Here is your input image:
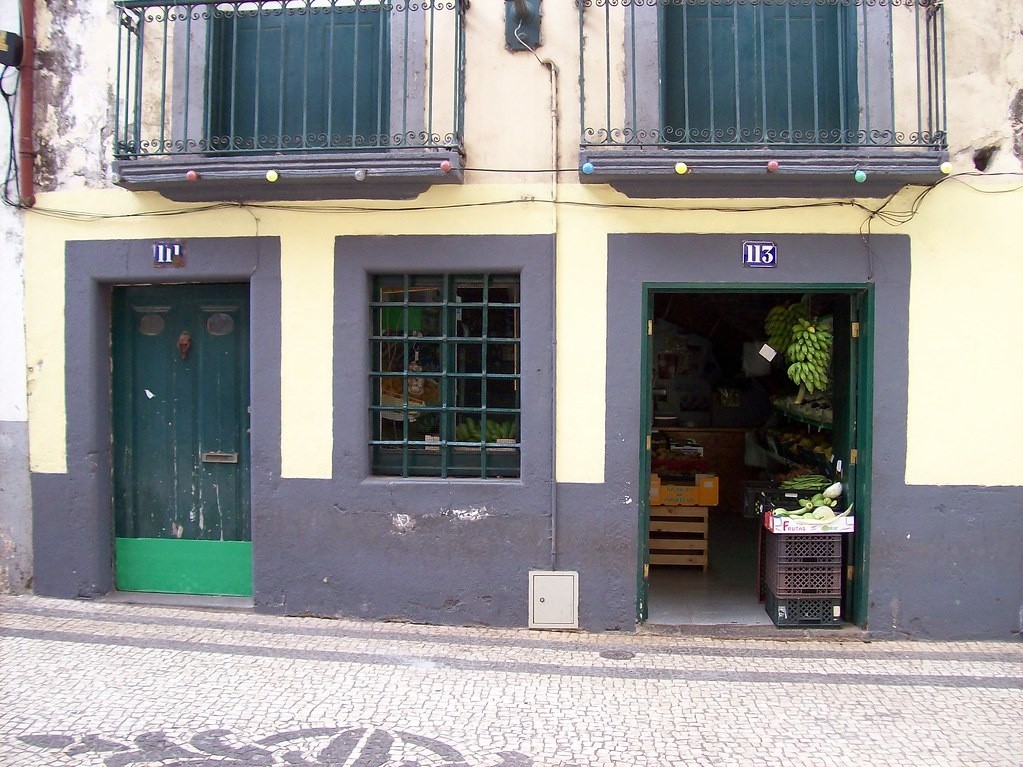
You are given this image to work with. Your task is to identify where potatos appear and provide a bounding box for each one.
[651,447,704,461]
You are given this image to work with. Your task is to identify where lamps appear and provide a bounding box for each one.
[177,330,193,361]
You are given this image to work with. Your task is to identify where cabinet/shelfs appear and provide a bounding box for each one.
[761,412,834,476]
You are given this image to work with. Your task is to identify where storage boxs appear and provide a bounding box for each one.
[651,438,709,480]
[765,432,833,474]
[762,512,855,630]
[736,481,843,519]
[650,472,719,507]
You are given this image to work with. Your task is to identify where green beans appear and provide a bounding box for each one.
[778,476,829,491]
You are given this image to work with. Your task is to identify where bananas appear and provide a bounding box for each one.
[765,304,833,394]
[453,416,517,440]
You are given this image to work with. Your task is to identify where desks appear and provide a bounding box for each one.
[653,426,753,499]
